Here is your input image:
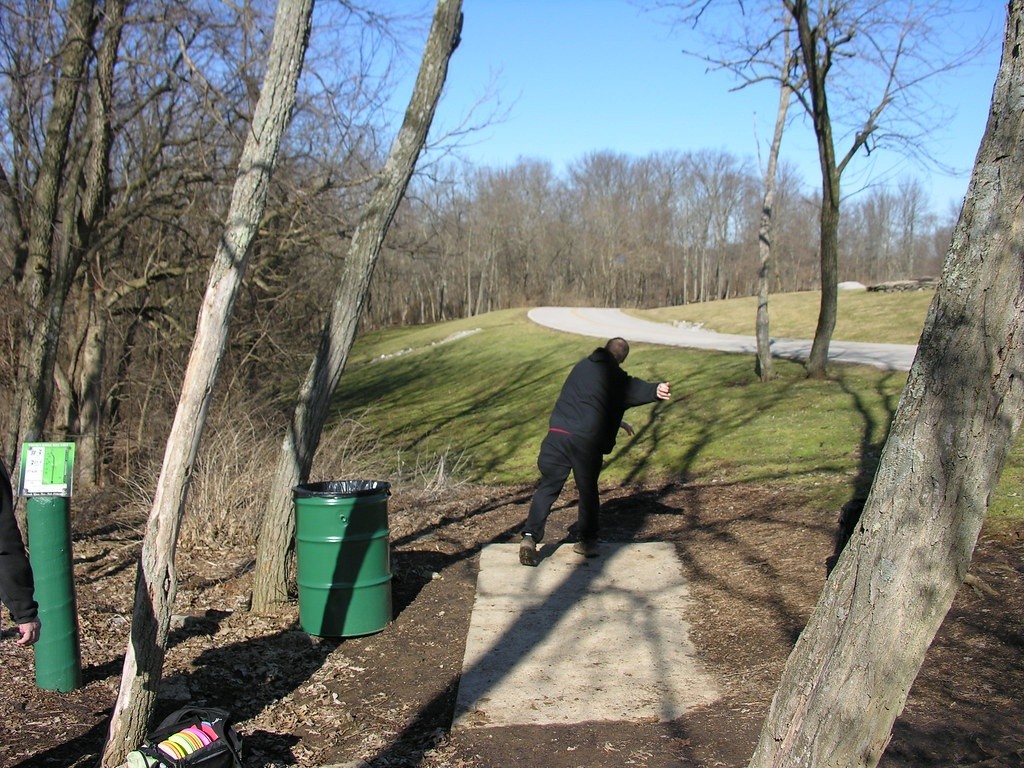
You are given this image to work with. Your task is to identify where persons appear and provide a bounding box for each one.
[0,459,42,646]
[520,338,671,567]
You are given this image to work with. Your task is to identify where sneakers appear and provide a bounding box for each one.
[519,536,538,567]
[572,542,601,559]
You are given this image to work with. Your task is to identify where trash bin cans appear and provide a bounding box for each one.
[293,480,394,636]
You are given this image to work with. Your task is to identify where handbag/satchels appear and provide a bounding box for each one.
[126,705,243,768]
